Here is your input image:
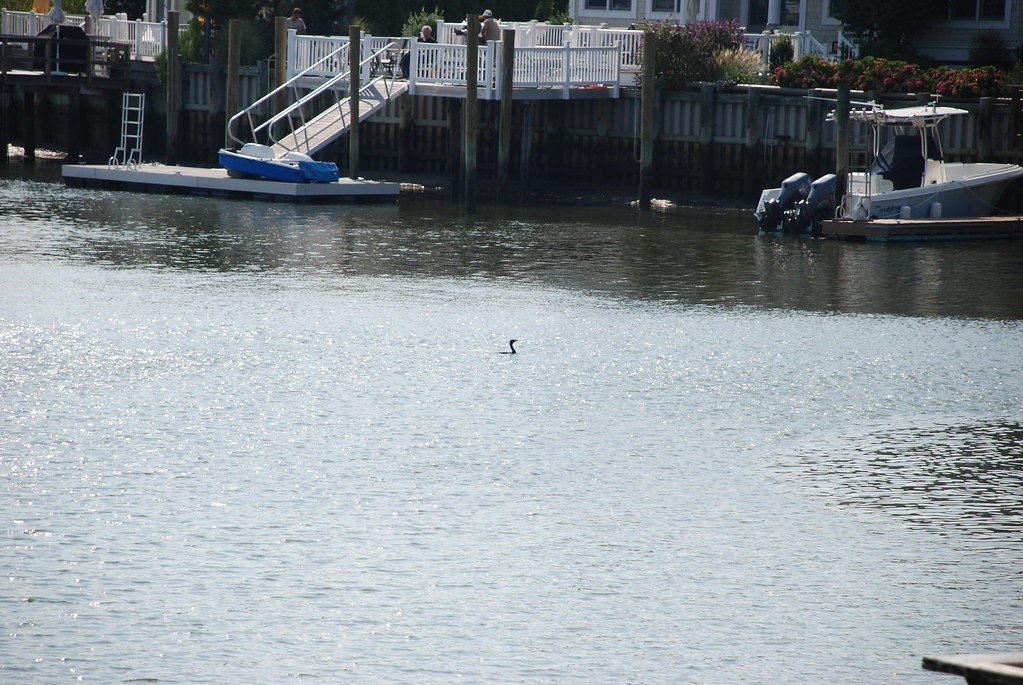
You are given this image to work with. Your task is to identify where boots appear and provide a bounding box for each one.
[453,28,462,35]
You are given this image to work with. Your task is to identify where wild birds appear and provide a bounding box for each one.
[498,339,519,354]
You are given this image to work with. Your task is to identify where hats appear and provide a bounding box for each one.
[482,9,491,16]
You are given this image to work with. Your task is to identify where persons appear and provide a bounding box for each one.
[286,7,306,34]
[398,24,440,81]
[481,9,501,41]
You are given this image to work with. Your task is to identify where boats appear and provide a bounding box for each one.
[755,105,1022,218]
[217,142,340,183]
